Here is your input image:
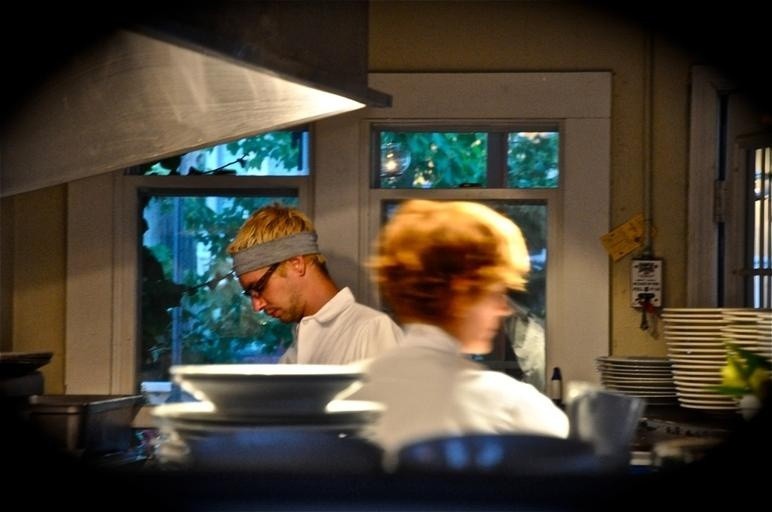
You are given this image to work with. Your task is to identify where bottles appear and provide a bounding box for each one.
[551,367,561,404]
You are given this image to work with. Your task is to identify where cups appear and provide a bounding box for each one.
[564,379,648,465]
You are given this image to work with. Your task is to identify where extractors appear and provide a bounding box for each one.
[0,1,392,201]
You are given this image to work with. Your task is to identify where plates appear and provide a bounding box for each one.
[150,363,386,473]
[595,307,772,411]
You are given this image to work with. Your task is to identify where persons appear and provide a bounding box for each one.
[336,198,571,472]
[226,202,404,365]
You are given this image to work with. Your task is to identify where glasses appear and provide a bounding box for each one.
[242,263,280,297]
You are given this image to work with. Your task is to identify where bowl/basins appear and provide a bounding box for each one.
[398,434,595,512]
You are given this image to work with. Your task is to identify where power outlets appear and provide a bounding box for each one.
[631,283,664,309]
